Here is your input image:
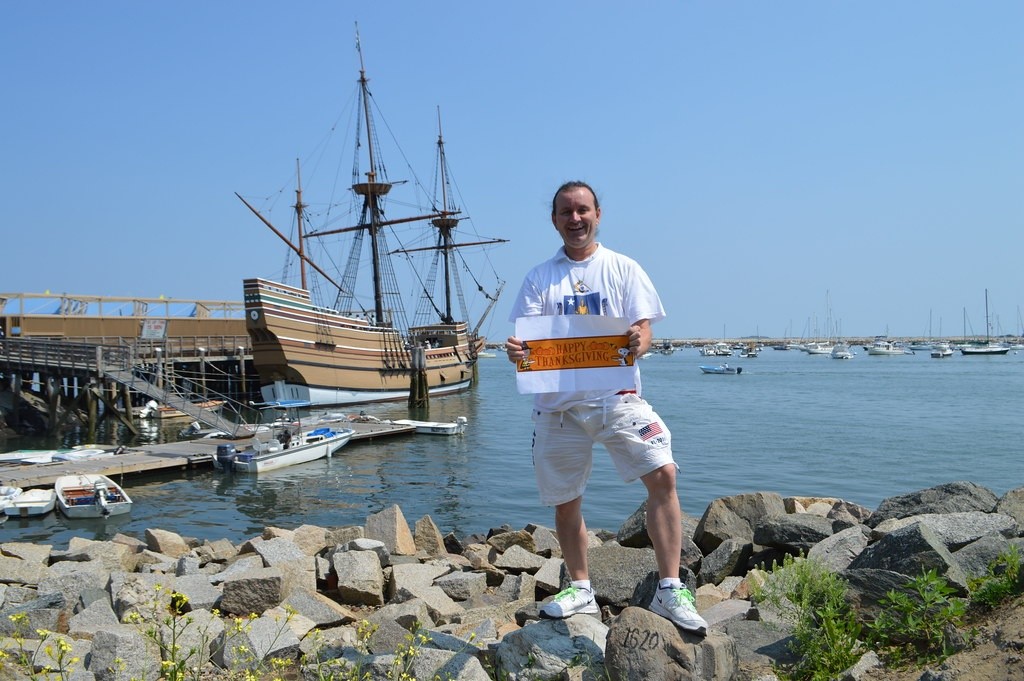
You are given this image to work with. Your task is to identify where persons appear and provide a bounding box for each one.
[507,180,708,635]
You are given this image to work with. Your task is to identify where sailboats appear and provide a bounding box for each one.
[771,289,854,359]
[863,289,1024,357]
[234,20,509,409]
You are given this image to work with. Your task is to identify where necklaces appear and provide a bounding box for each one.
[564,255,594,293]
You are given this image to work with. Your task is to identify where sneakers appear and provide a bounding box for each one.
[648,582,707,637]
[538,587,598,619]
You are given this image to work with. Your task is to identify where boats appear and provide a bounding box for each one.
[210,400,356,473]
[699,342,764,358]
[0,486,23,513]
[699,366,742,375]
[4,488,56,515]
[55,474,133,518]
[270,413,469,435]
[0,449,114,463]
